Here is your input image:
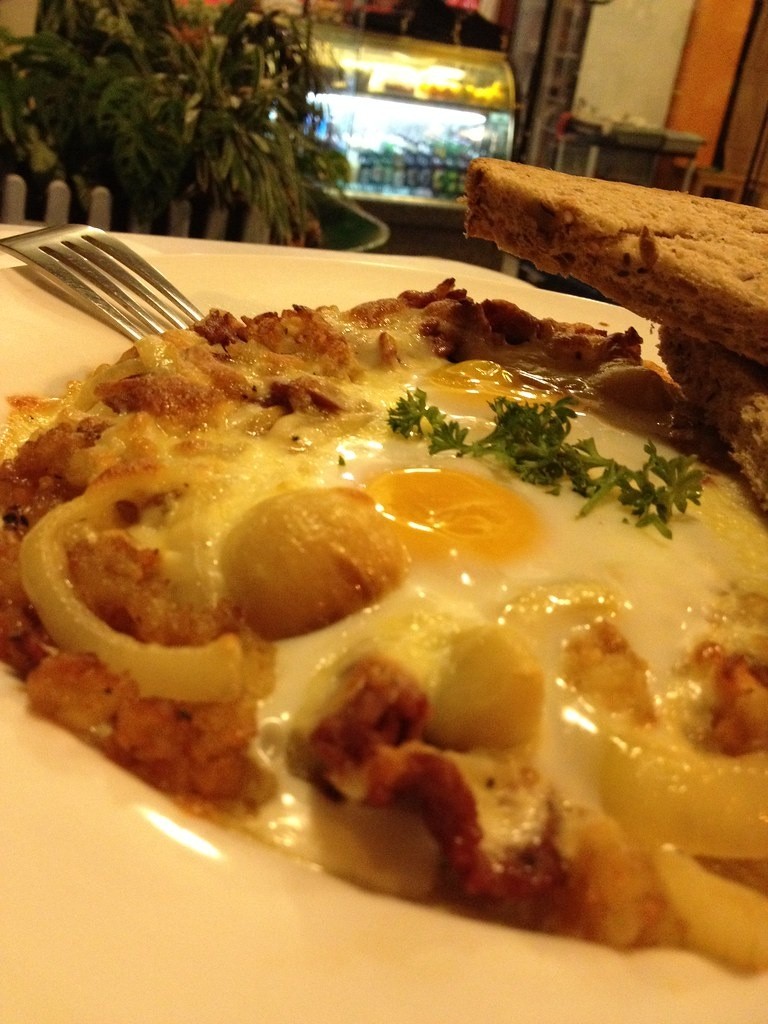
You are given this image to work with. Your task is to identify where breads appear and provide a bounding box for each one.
[464,154,768,517]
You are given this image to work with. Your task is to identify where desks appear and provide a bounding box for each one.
[556,117,707,193]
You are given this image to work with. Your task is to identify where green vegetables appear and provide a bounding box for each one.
[380,385,711,541]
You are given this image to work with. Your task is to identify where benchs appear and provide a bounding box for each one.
[669,161,747,203]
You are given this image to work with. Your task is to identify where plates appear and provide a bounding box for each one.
[0,237,767,1024]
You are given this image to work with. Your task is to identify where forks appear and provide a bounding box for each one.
[0,221,206,342]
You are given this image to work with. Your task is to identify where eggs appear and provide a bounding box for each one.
[361,460,567,587]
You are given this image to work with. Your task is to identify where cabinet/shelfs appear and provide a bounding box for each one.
[245,11,517,270]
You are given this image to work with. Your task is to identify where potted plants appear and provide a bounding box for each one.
[0,0,394,256]
